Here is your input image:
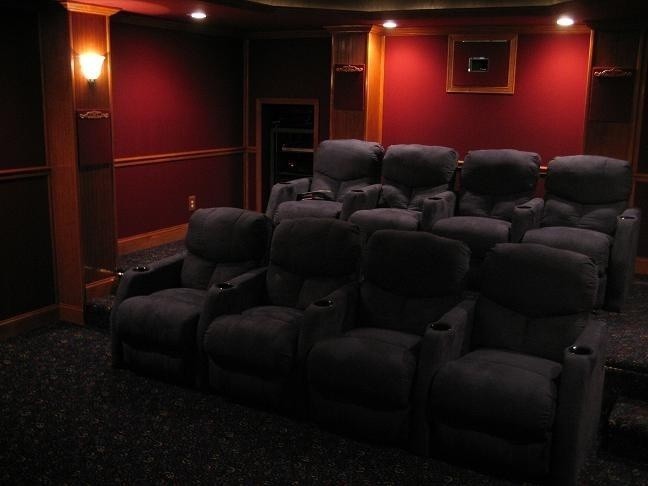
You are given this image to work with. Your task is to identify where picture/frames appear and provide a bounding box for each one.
[446,31,518,94]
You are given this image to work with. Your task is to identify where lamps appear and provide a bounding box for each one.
[77,52,105,88]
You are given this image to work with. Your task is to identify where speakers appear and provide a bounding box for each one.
[333,64,365,113]
[588,66,633,122]
